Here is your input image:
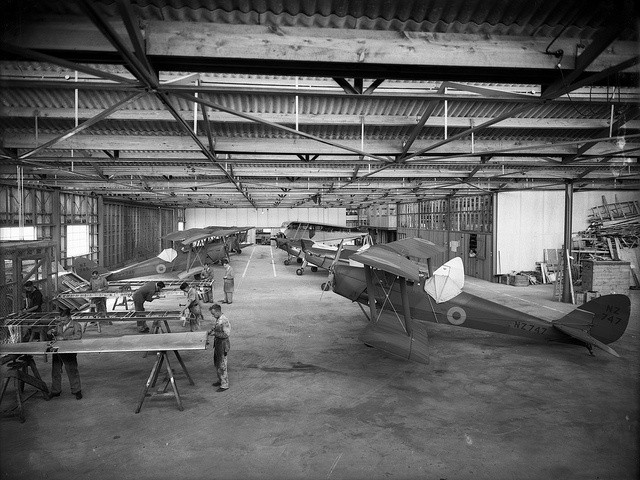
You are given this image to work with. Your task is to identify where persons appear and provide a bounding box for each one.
[48,307,83,400]
[86,271,110,324]
[206,304,231,392]
[222,259,234,305]
[200,263,214,304]
[22,280,44,341]
[178,283,203,333]
[132,281,166,333]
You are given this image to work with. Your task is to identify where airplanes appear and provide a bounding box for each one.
[271,221,374,275]
[72,228,228,281]
[160,226,253,255]
[328,237,631,365]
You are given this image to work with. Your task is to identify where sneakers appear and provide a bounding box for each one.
[217,387,229,392]
[48,392,61,399]
[213,382,221,386]
[76,390,82,400]
